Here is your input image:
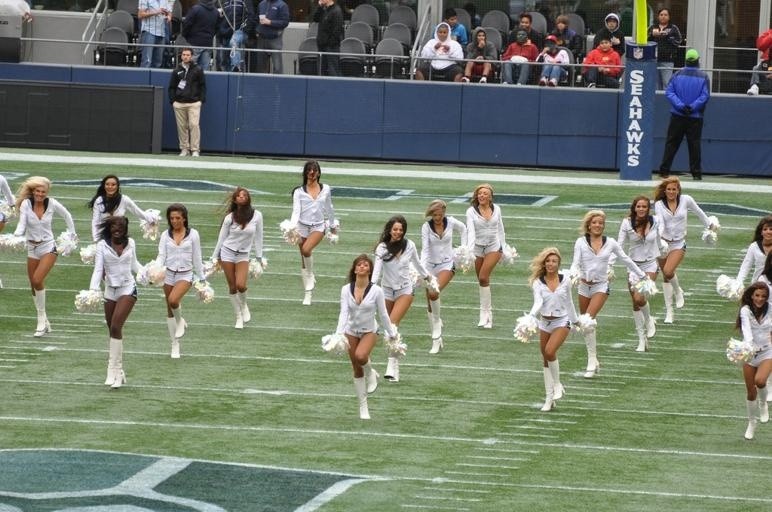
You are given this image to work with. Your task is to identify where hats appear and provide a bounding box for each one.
[684,48,700,62]
[543,35,559,45]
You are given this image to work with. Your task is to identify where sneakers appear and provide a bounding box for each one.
[746,83,759,95]
[459,76,560,87]
[177,150,201,157]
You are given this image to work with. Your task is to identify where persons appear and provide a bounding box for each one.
[79,176,161,280]
[321,184,520,420]
[512,175,721,412]
[280,161,340,305]
[415,0,683,91]
[168,47,207,157]
[311,0,343,77]
[203,187,266,330]
[747,29,772,95]
[149,205,215,359]
[75,214,149,388]
[660,49,710,180]
[0,174,17,288]
[135,0,290,73]
[715,216,772,440]
[0,176,78,337]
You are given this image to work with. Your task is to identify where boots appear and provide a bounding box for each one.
[638,300,656,338]
[667,274,684,309]
[428,297,443,340]
[477,285,493,329]
[546,359,565,401]
[662,282,673,324]
[540,366,556,412]
[353,375,371,420]
[582,324,600,379]
[361,357,380,395]
[757,386,769,423]
[31,254,317,388]
[383,354,399,382]
[744,398,757,440]
[427,310,444,354]
[632,308,649,352]
